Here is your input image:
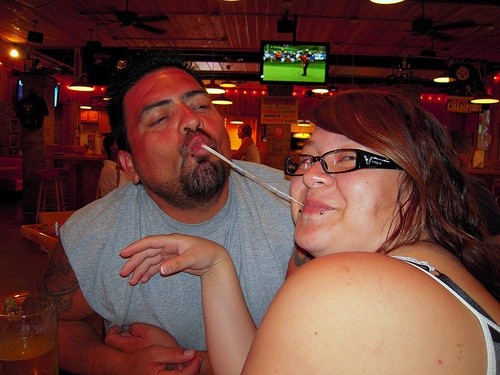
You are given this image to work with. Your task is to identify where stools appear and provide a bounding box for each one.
[33,168,65,225]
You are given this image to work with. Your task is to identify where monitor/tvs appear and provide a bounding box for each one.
[259,40,329,85]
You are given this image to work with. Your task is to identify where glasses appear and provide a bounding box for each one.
[283,148,411,176]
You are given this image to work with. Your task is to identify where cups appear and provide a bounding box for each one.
[0,294,59,375]
[50,217,65,238]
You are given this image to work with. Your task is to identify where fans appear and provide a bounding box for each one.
[401,0,478,43]
[89,0,171,35]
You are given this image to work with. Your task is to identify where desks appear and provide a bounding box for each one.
[41,151,105,210]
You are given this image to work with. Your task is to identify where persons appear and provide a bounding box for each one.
[263,47,326,64]
[114,83,500,375]
[94,133,136,204]
[298,51,310,76]
[228,123,260,164]
[14,45,330,374]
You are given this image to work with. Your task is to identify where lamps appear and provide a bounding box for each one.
[205,71,236,105]
[66,47,95,91]
[433,60,456,84]
[470,63,500,104]
